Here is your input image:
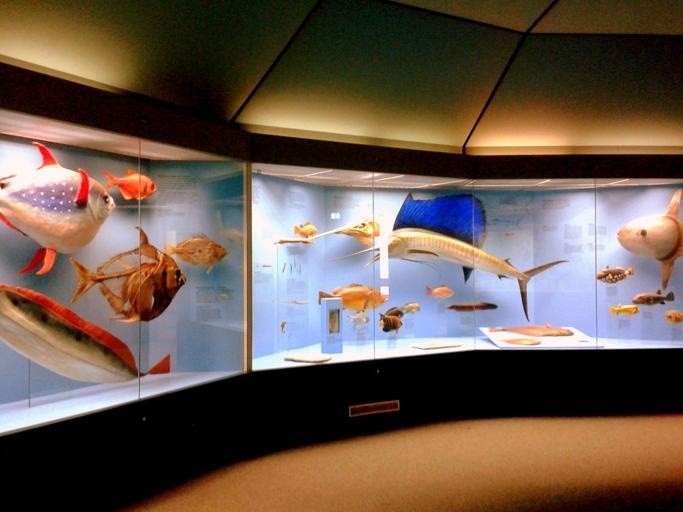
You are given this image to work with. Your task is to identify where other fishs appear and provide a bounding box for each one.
[1,141,683,382]
